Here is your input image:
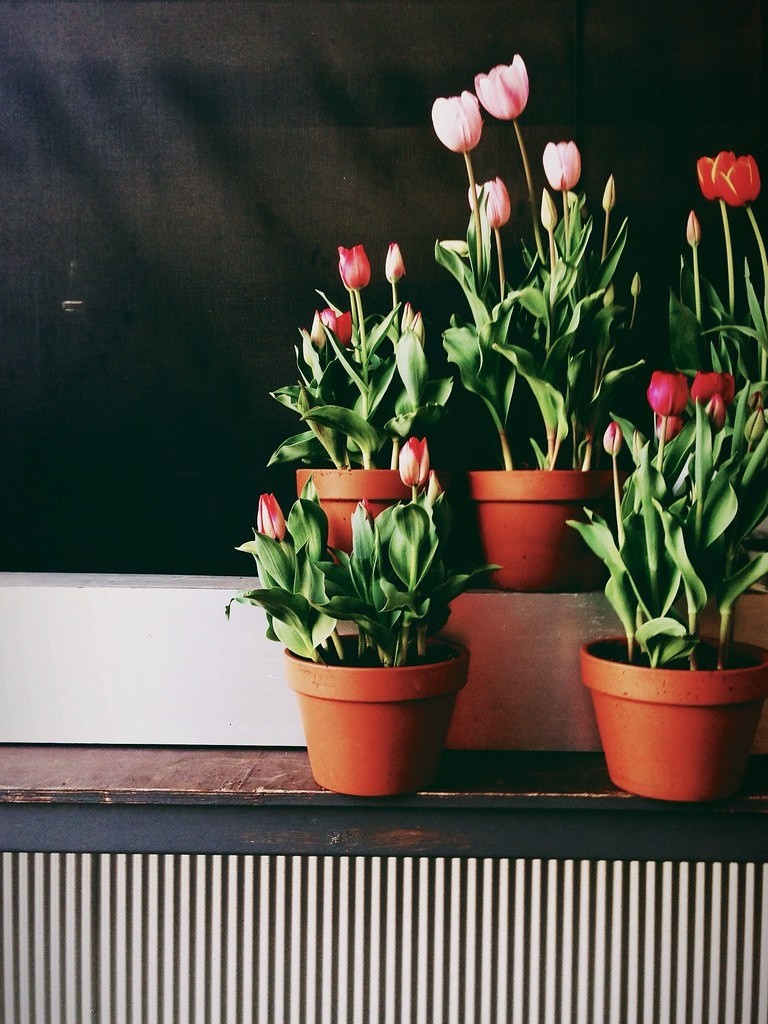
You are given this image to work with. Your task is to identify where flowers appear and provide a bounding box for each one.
[659,152,767,381]
[562,370,768,670]
[225,436,501,667]
[430,55,643,472]
[266,242,452,469]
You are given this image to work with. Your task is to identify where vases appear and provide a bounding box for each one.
[468,471,628,592]
[578,633,767,801]
[282,632,470,796]
[295,469,427,565]
